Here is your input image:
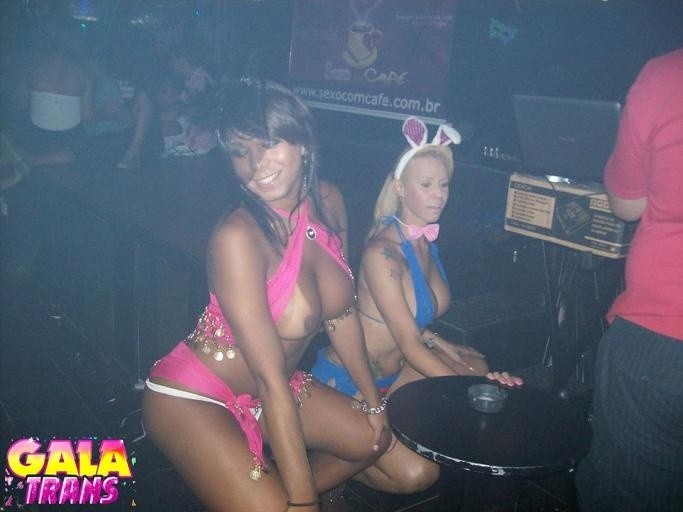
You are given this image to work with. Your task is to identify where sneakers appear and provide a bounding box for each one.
[113,147,144,171]
[166,141,210,157]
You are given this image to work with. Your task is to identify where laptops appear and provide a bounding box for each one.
[510,91,623,188]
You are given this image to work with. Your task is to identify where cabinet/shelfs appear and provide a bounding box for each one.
[501,226,630,369]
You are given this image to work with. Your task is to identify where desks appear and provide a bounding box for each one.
[385,375,593,512]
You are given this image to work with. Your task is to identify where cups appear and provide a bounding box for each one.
[349,21,383,59]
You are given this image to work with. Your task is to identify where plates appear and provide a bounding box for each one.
[341,49,379,68]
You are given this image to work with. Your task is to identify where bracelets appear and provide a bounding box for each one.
[427,330,440,347]
[285,499,322,508]
[357,393,390,420]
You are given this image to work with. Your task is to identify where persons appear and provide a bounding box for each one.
[309,108,523,497]
[139,74,400,512]
[0,0,222,176]
[572,46,683,512]
